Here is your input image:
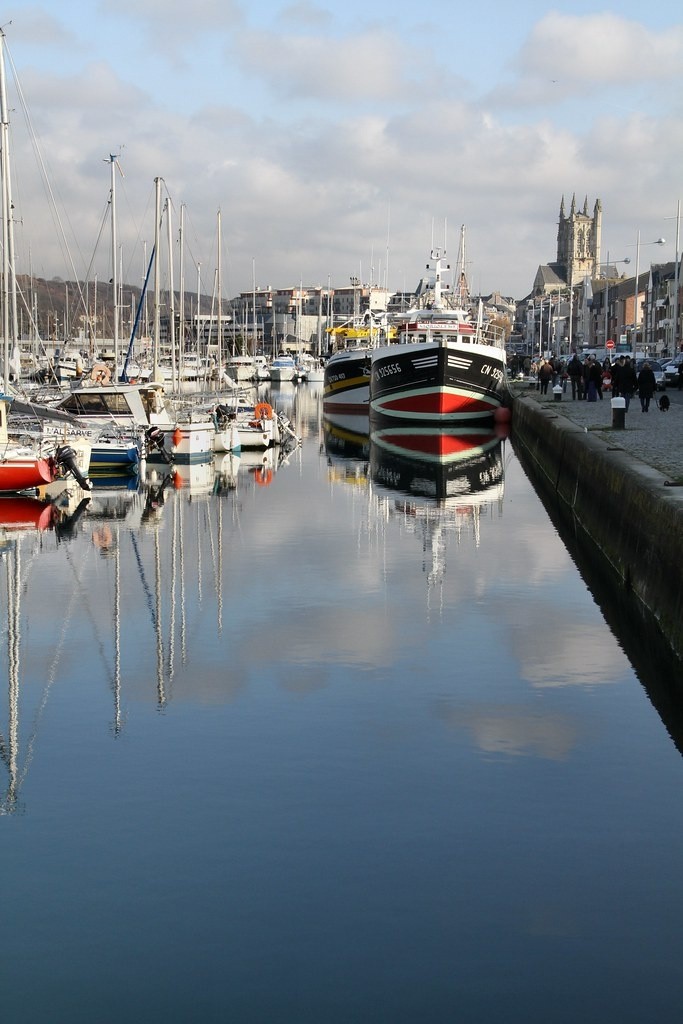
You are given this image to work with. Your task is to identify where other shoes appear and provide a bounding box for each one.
[645,409,648,412]
[642,406,646,412]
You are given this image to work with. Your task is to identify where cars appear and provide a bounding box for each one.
[634,359,666,392]
[558,355,574,365]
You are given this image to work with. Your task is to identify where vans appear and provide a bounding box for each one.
[598,353,649,367]
[657,352,683,386]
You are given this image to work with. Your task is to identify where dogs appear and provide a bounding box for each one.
[659,395,670,412]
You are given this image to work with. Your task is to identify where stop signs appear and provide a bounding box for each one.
[606,340,614,349]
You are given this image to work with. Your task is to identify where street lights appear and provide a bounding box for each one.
[578,267,606,348]
[526,279,582,356]
[595,251,632,349]
[626,230,666,358]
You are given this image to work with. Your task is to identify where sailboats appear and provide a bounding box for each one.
[1,448,284,816]
[0,21,319,515]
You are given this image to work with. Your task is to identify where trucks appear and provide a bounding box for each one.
[579,349,616,365]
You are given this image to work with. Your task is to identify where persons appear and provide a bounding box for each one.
[206,403,229,434]
[506,352,569,395]
[567,353,657,413]
[427,300,432,310]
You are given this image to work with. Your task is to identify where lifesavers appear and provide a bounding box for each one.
[173,429,182,447]
[255,469,273,485]
[90,364,111,385]
[255,403,273,419]
[174,470,182,489]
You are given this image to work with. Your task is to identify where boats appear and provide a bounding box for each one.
[369,416,507,622]
[321,192,396,407]
[368,217,509,424]
[320,404,389,585]
